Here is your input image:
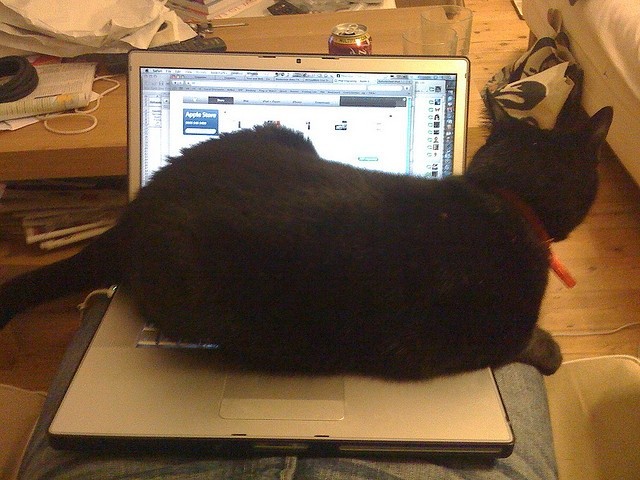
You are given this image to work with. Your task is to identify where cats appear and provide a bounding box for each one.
[2,87,613,383]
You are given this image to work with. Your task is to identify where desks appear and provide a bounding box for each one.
[0,5,450,180]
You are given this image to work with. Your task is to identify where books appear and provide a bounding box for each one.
[165,0,260,23]
[0,61,96,123]
[2,178,128,251]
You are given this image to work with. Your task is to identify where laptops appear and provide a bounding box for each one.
[42,49,530,466]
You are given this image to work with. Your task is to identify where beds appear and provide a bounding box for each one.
[522,0,640,188]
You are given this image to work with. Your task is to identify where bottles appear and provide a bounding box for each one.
[327,21,372,55]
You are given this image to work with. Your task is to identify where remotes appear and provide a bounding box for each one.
[103,36,228,71]
